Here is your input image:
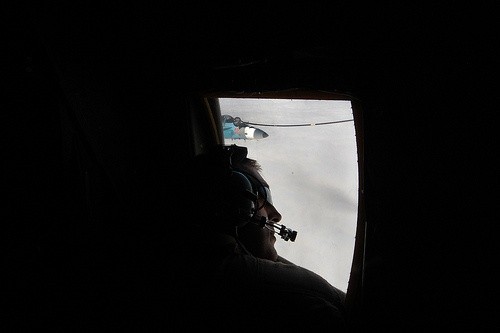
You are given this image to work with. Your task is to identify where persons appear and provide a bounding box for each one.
[167,149,346,333]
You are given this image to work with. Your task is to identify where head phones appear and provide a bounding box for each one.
[214,144,255,223]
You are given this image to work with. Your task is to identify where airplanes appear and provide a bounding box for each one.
[222,115,269,142]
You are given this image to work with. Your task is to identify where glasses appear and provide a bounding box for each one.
[255,186,274,207]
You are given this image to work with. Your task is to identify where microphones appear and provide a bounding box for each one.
[252,214,297,243]
